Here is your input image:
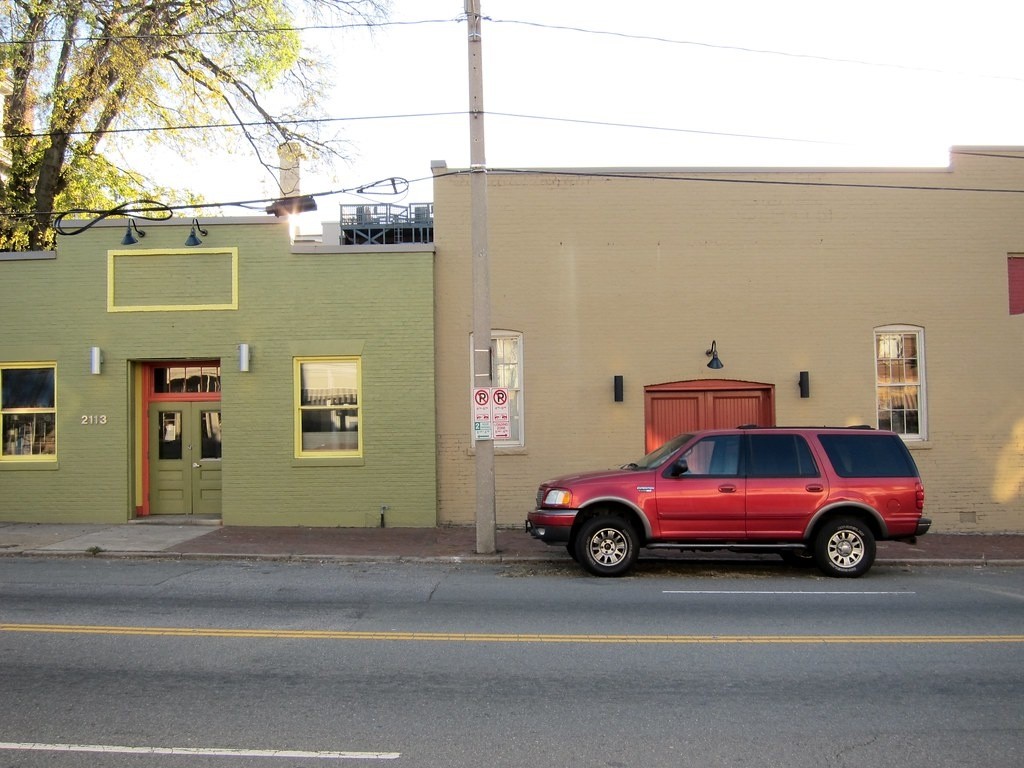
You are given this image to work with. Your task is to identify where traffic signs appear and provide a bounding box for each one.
[473,386,493,440]
[490,387,511,439]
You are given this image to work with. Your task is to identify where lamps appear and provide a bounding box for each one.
[239,343,251,371]
[121,218,145,246]
[614,376,624,402]
[799,371,809,398]
[706,340,724,369]
[185,218,208,246]
[91,346,103,374]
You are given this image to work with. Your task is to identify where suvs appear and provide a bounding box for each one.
[524,424,932,578]
